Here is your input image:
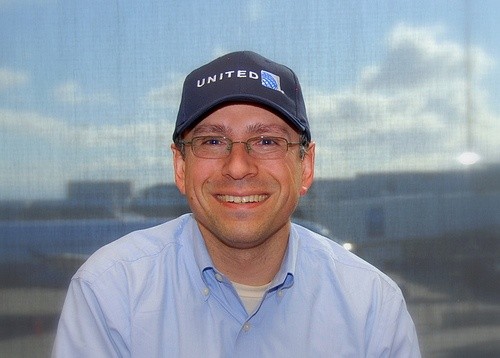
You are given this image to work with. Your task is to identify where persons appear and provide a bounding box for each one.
[52,49,422,358]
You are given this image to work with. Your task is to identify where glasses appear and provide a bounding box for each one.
[175,135,307,159]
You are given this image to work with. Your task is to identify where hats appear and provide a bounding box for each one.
[171,50,314,144]
